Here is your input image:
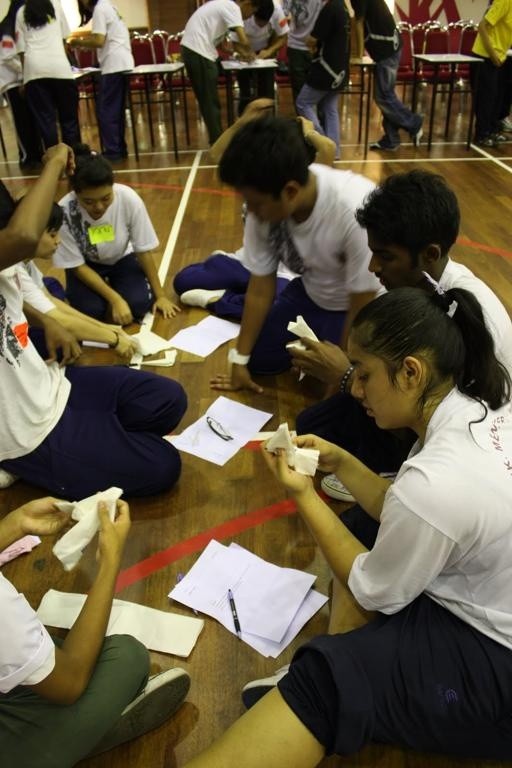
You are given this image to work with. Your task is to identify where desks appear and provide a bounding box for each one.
[126,63,192,161]
[74,67,104,159]
[220,58,279,128]
[412,53,486,152]
[347,57,378,160]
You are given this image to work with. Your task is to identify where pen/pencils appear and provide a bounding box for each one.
[228,589,242,640]
[134,318,146,325]
[113,363,138,367]
[177,573,199,615]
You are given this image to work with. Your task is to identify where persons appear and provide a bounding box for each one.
[0,0,512,172]
[167,97,511,504]
[2,146,189,500]
[0,472,191,766]
[178,287,511,766]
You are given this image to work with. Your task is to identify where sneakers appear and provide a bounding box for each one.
[475,135,499,147]
[86,666,192,758]
[180,289,226,309]
[369,140,401,152]
[491,131,511,144]
[240,662,296,708]
[103,147,129,162]
[320,465,405,505]
[411,116,424,147]
[498,116,512,128]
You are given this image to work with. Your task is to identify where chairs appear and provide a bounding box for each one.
[215,41,291,87]
[364,19,479,112]
[64,30,192,126]
